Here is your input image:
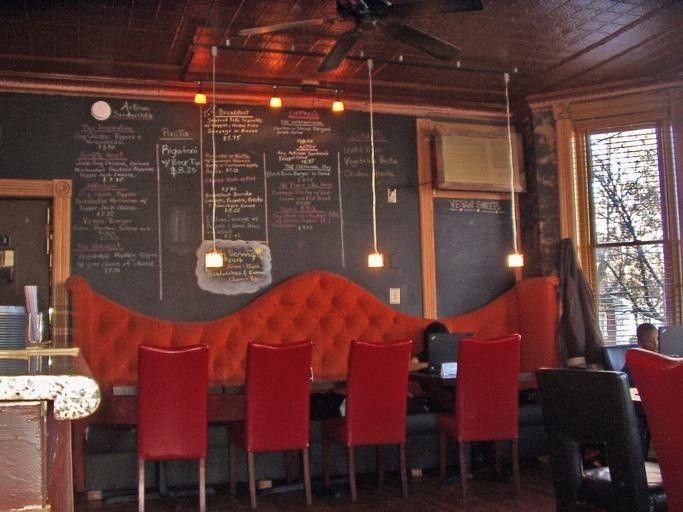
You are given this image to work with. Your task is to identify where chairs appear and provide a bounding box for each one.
[439,336,521,504]
[136,341,211,512]
[225,338,313,512]
[536,343,682,511]
[322,337,417,502]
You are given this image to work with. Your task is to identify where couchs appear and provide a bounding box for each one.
[66,276,562,495]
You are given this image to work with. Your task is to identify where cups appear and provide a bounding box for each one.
[24,313,43,345]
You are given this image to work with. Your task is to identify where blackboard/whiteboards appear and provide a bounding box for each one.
[0,79,523,320]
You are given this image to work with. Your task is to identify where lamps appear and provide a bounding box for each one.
[201,46,227,272]
[501,71,525,270]
[364,55,389,270]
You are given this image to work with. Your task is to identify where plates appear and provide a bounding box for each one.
[0,304,26,377]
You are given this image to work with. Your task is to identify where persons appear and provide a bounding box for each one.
[409,322,511,479]
[620,323,660,463]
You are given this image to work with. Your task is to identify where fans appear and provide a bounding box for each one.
[240,1,486,75]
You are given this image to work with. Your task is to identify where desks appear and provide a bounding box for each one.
[71,370,532,512]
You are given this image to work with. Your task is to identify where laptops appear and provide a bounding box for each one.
[422,332,474,373]
[658,325,683,357]
